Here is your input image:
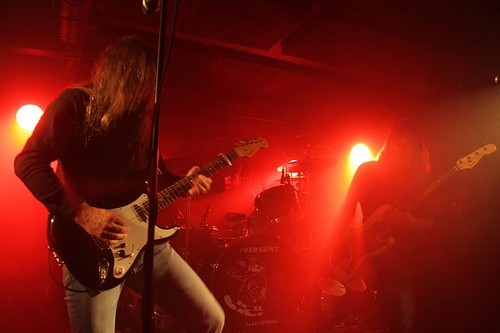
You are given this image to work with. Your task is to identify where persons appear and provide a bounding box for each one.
[325,121,439,330]
[13,33,226,333]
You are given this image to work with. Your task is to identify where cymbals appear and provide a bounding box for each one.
[155,156,237,199]
[275,156,339,173]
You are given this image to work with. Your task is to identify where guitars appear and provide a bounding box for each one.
[46,135,267,291]
[327,144,497,282]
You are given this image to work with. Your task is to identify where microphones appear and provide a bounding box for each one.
[280,167,285,184]
[202,205,211,225]
[142,0,161,14]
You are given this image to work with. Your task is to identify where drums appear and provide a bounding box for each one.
[198,234,309,326]
[252,183,308,233]
[165,225,219,258]
